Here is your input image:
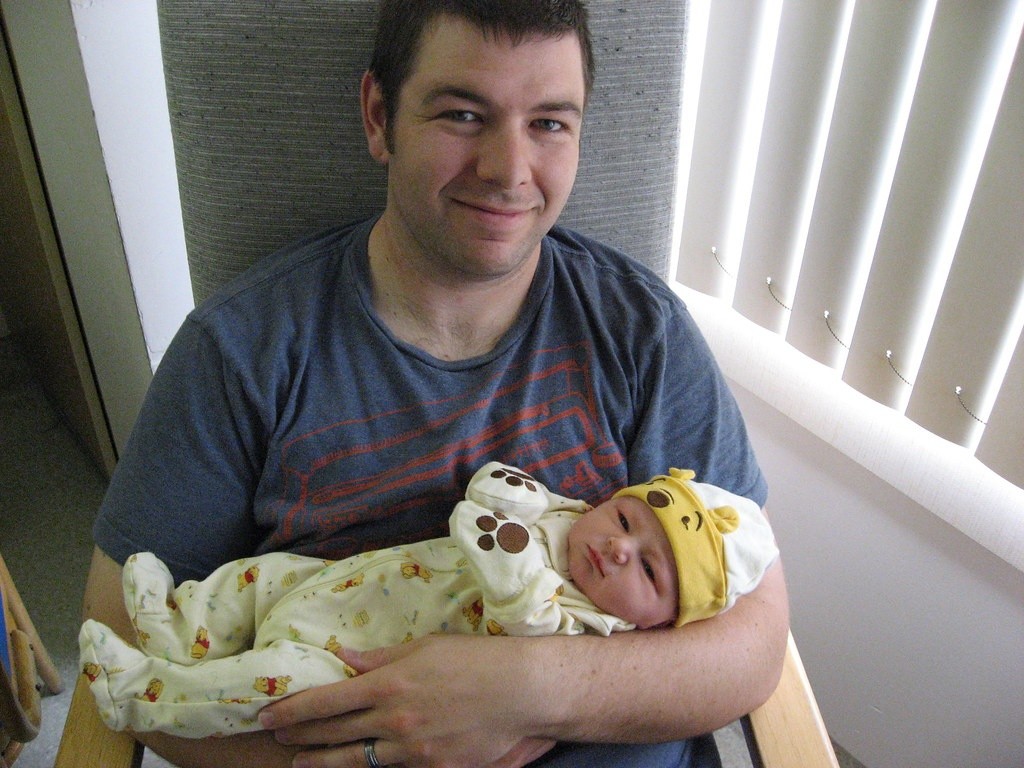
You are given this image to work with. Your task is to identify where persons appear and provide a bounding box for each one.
[81,0,792,768]
[76,458,779,738]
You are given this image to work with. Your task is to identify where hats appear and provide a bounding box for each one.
[612,467,778,628]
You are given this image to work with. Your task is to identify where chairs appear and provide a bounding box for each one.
[57,3,839,768]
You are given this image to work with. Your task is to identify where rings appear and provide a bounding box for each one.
[364,743,379,768]
